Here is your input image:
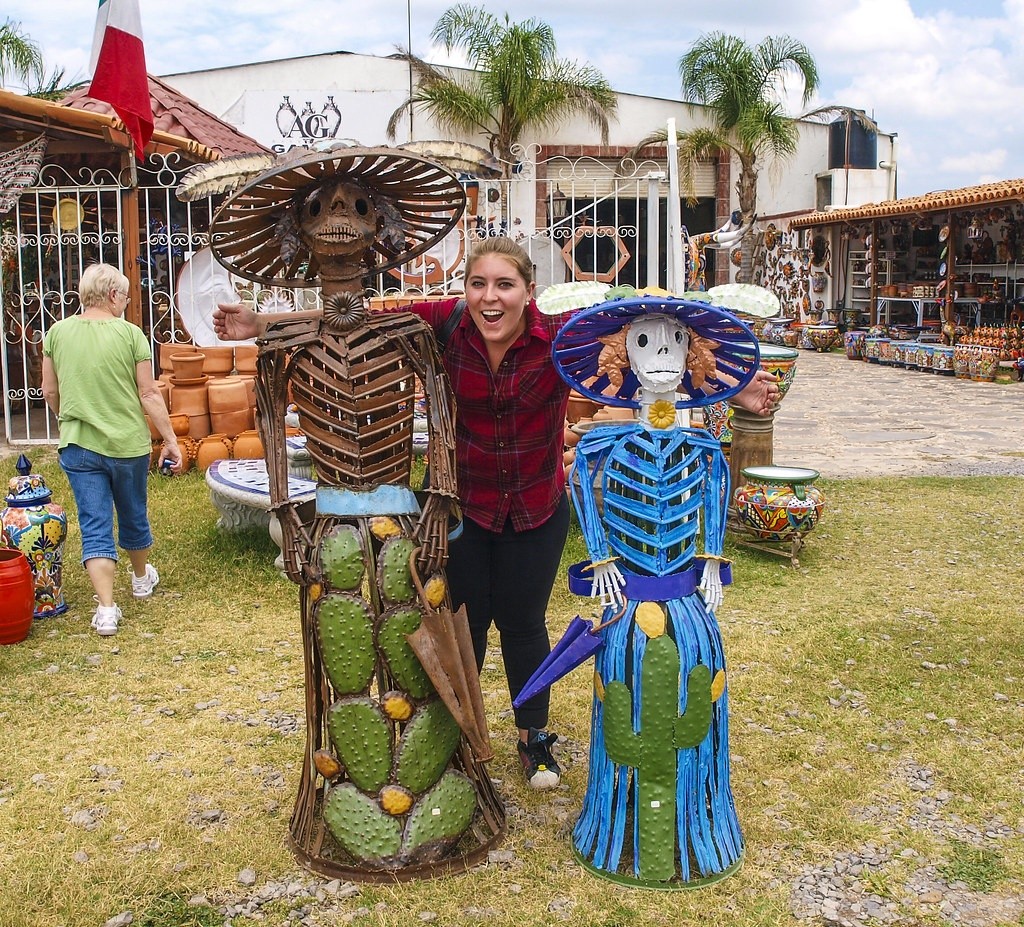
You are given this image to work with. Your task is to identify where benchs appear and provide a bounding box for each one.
[205,457,315,538]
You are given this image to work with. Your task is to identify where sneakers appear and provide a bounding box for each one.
[517,727,561,792]
[91,594,122,635]
[127,563,159,600]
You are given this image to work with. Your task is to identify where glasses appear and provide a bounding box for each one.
[118,290,132,304]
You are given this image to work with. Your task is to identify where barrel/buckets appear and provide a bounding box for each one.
[0,548,36,645]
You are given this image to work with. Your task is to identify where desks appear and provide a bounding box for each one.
[877,297,983,332]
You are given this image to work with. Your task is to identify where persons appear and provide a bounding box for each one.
[42,263,183,635]
[212,237,779,792]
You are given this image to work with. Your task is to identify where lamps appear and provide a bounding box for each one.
[542,182,569,226]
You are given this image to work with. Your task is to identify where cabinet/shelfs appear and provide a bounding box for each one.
[955,258,1010,323]
[1014,259,1024,322]
[849,250,908,329]
[915,249,941,285]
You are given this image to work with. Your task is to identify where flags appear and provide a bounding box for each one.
[87,0,154,165]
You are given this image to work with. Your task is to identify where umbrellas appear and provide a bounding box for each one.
[512,594,627,708]
[405,547,496,763]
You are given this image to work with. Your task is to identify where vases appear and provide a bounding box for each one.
[146,341,256,473]
[731,466,827,547]
[812,271,826,293]
[741,317,1001,405]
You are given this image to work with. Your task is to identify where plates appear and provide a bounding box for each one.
[866,235,878,247]
[865,277,877,290]
[865,250,871,261]
[865,263,878,276]
[940,247,947,259]
[937,280,946,291]
[939,262,946,276]
[938,226,950,242]
